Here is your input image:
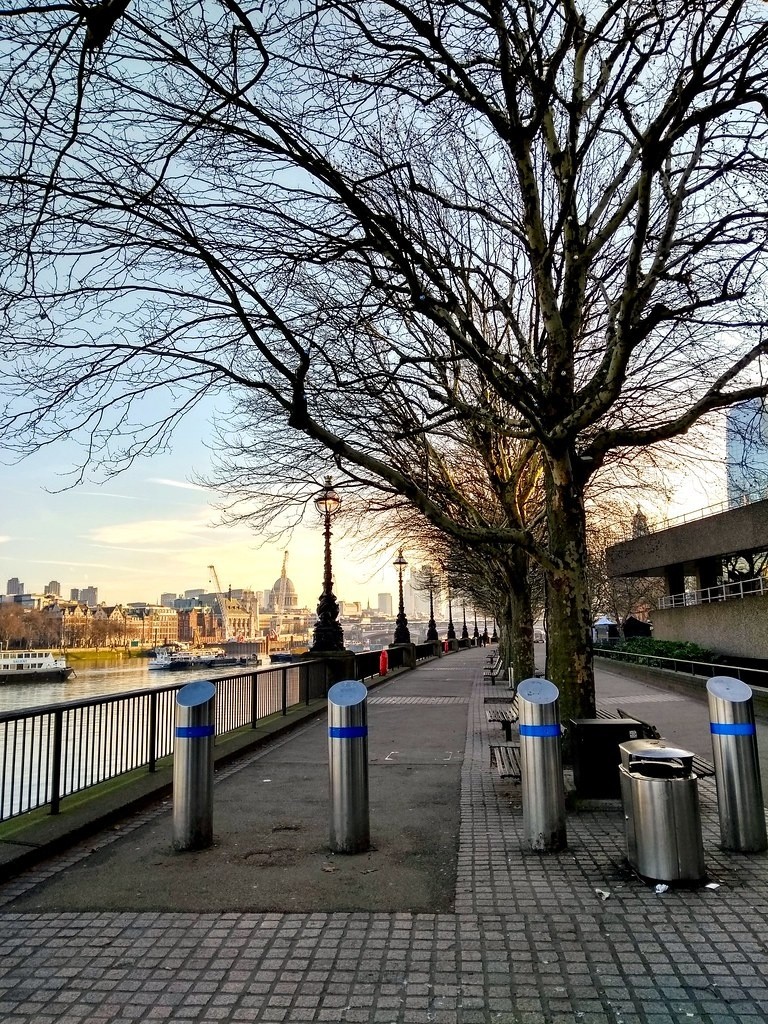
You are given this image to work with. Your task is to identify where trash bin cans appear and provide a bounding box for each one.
[617,740,707,885]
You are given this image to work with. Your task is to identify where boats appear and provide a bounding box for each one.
[0,641,77,684]
[146,647,262,671]
[269,654,301,662]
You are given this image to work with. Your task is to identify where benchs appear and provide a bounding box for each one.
[535,671,545,679]
[485,692,519,743]
[488,722,569,781]
[595,707,621,720]
[483,655,501,673]
[486,648,499,664]
[616,707,715,778]
[483,659,503,685]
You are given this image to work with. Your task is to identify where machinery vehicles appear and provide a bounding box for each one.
[207,565,237,644]
[266,550,289,651]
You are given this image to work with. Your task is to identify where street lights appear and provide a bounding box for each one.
[445,575,459,650]
[389,546,416,667]
[460,599,471,647]
[423,566,441,656]
[299,474,359,699]
[472,609,481,645]
